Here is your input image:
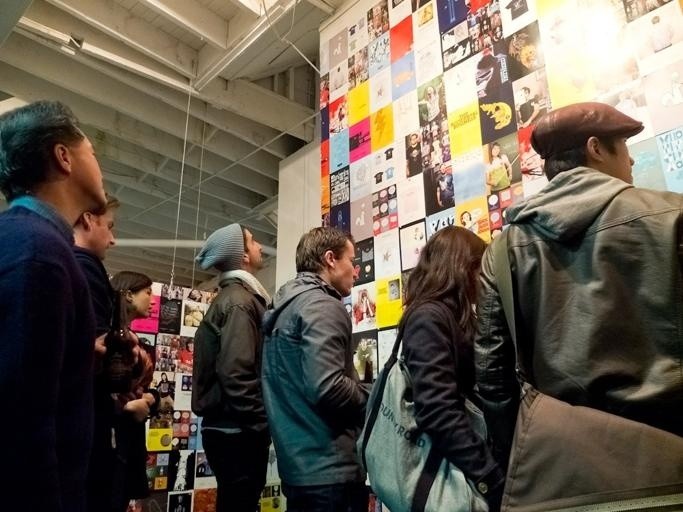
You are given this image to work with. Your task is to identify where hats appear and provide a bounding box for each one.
[195,223,244,272]
[531,102,645,159]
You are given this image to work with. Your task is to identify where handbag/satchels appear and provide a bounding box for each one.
[501,382,683,512]
[356,358,489,512]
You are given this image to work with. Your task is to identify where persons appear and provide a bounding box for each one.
[192,222,277,512]
[138,285,215,512]
[0,99,139,510]
[262,228,378,512]
[107,271,162,512]
[71,190,156,511]
[401,225,503,502]
[319,0,672,381]
[473,102,683,512]
